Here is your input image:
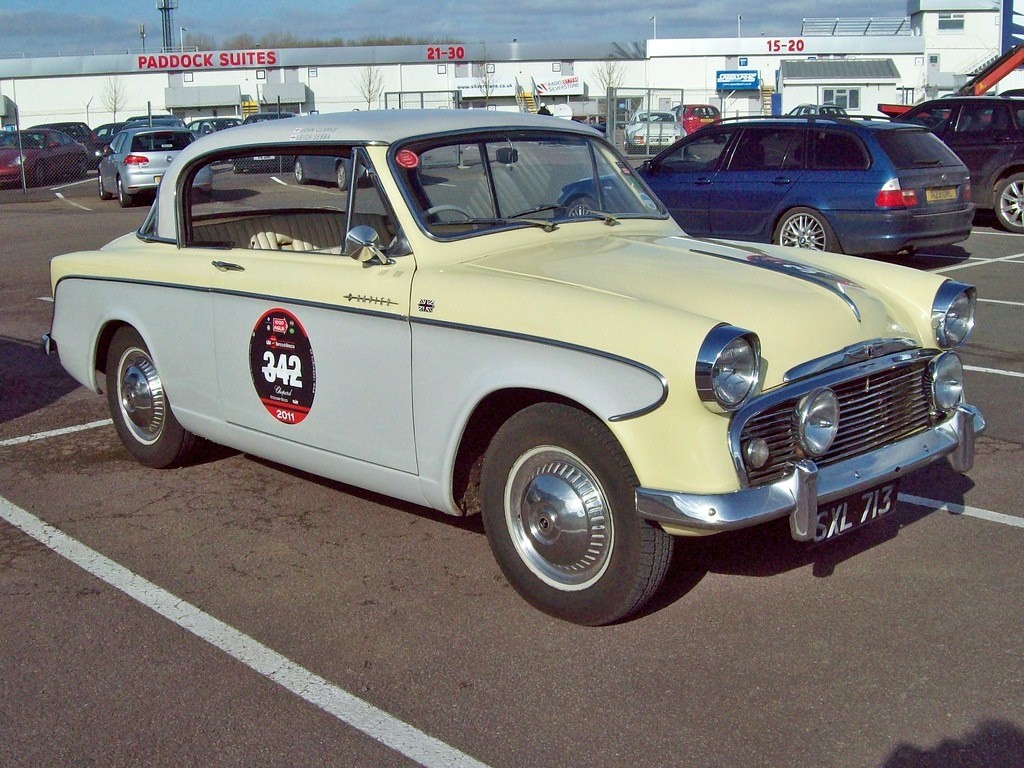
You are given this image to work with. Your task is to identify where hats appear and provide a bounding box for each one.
[540,103,545,107]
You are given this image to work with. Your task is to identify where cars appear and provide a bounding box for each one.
[292,155,373,191]
[38,108,985,625]
[671,104,721,134]
[186,117,242,135]
[94,126,213,208]
[92,123,132,144]
[623,109,688,153]
[233,113,300,174]
[24,122,100,168]
[0,128,89,191]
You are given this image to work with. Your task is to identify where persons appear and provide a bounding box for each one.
[537,102,553,116]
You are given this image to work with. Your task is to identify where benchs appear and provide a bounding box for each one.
[191,208,391,252]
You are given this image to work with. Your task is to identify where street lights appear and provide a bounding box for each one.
[738,14,742,38]
[181,26,188,52]
[650,15,656,39]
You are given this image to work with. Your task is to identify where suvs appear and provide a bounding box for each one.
[893,95,1024,234]
[554,116,977,257]
[784,102,850,120]
